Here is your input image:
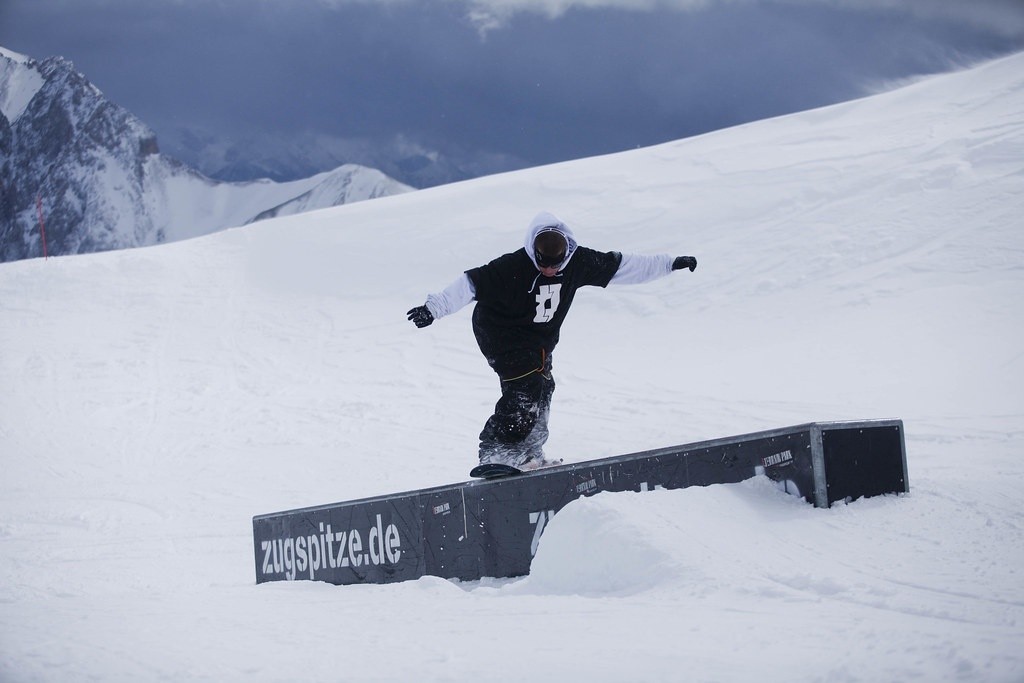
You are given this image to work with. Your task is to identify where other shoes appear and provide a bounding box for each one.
[517,456,561,471]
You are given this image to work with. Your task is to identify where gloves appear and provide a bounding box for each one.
[407,305,433,328]
[671,256,696,273]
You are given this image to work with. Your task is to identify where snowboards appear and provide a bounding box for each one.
[468,457,564,479]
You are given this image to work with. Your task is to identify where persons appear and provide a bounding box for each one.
[406,211,697,469]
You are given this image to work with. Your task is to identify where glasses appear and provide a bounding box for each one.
[534,248,566,269]
